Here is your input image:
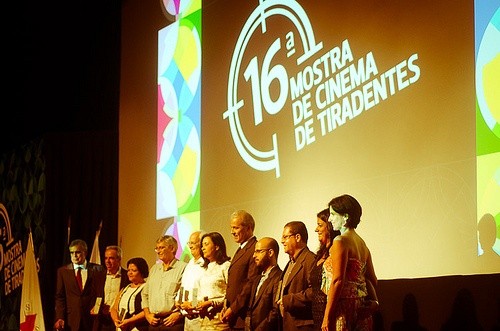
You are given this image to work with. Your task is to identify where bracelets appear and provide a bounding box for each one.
[369,299,380,306]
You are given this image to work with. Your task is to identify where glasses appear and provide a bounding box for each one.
[187,242,199,246]
[154,247,166,253]
[283,234,296,239]
[252,248,271,254]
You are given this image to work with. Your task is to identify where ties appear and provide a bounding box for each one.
[76,268,83,292]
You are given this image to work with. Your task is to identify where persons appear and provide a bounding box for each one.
[321,193,378,331]
[193,231,233,331]
[275,220,320,331]
[219,209,260,331]
[174,230,211,331]
[91,245,131,331]
[142,234,188,331]
[242,237,284,331]
[315,206,380,331]
[54,238,102,331]
[114,257,150,331]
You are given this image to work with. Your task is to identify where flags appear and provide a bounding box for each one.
[18,232,48,331]
